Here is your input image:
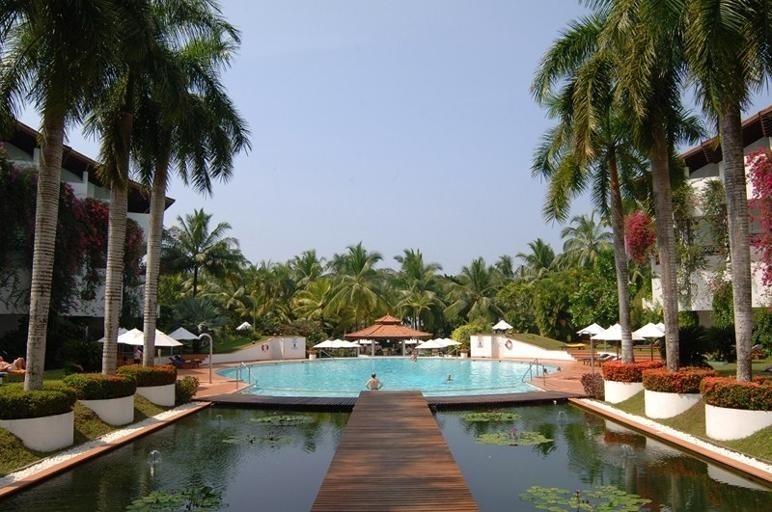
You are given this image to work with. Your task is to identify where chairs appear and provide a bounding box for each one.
[566,347,620,365]
[752,344,766,358]
[168,355,203,368]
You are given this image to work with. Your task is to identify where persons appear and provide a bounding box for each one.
[442,374,453,384]
[364,372,384,390]
[0,354,27,374]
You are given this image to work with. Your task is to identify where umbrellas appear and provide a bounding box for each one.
[310,338,380,356]
[397,335,463,356]
[236,321,252,331]
[573,321,665,361]
[97,325,201,368]
[490,320,515,331]
